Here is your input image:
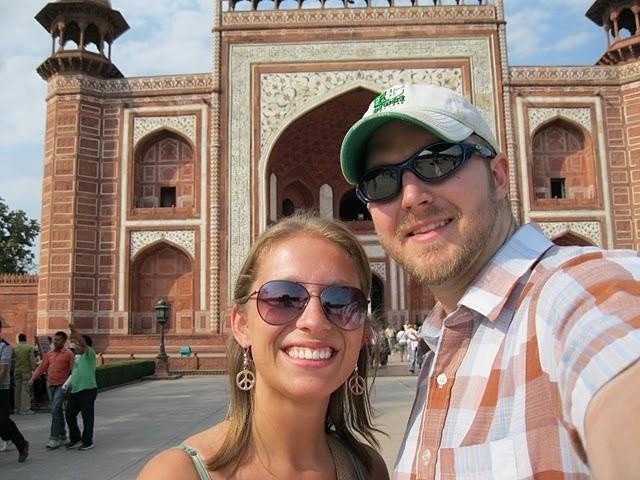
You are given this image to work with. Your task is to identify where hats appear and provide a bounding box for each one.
[339,83,501,185]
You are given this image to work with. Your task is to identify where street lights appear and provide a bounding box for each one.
[141,296,184,381]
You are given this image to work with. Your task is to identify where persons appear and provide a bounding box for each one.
[0,324,100,464]
[339,81,639,479]
[132,212,389,480]
[365,320,431,374]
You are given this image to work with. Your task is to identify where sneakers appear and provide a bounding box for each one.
[0,439,12,452]
[20,409,35,415]
[46,438,94,450]
[18,440,30,463]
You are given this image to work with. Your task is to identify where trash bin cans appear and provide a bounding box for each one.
[181,346,192,357]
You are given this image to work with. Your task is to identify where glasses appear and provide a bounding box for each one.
[355,141,497,204]
[237,281,370,331]
